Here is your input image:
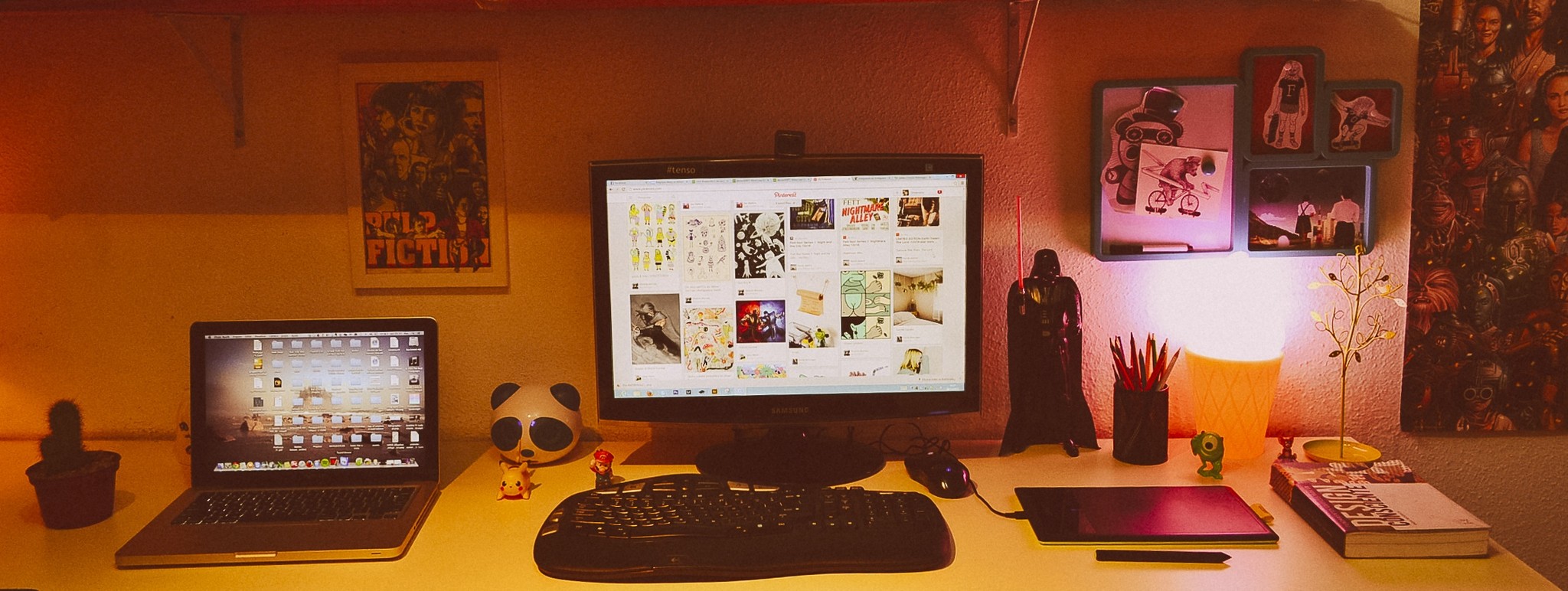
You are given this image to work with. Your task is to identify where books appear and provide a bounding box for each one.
[1274,459,1492,564]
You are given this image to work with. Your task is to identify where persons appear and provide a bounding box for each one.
[1409,0,1568,432]
[898,349,924,375]
[999,250,1102,459]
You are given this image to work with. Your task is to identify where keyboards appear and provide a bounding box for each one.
[531,471,956,586]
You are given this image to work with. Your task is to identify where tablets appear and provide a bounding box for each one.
[1014,481,1281,542]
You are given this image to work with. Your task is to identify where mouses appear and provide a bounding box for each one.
[903,441,969,499]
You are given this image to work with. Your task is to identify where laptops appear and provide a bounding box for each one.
[113,312,441,568]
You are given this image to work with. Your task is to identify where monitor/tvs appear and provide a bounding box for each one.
[584,153,984,427]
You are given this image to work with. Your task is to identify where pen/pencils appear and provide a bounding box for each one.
[1095,549,1233,563]
[1108,331,1182,391]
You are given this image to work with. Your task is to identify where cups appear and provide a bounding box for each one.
[1112,379,1169,466]
